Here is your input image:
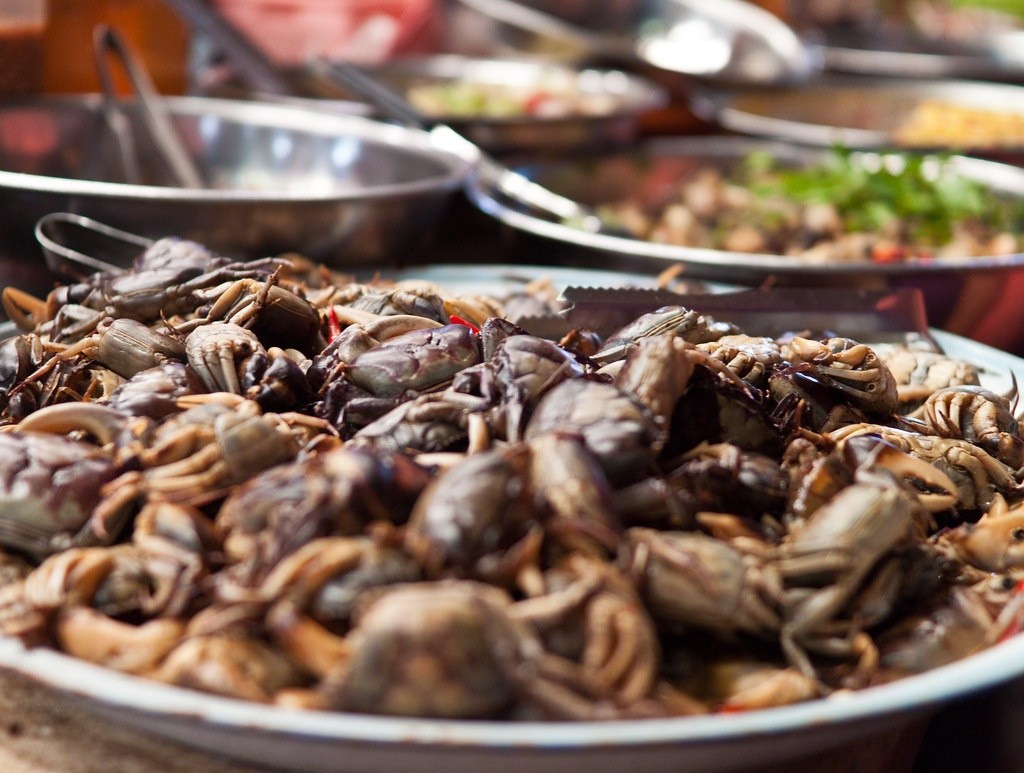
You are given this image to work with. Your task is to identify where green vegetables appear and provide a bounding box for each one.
[726,143,1024,252]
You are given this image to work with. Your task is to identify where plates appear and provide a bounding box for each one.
[0,259,1024,773]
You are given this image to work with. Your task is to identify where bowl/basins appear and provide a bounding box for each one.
[251,53,670,161]
[0,92,473,269]
[466,141,1024,349]
[689,71,1024,155]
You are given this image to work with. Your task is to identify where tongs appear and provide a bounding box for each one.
[33,212,157,278]
[88,20,206,199]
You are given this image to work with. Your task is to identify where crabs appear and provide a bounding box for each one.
[0,235,1024,718]
